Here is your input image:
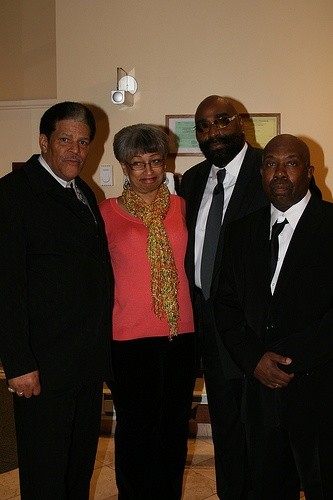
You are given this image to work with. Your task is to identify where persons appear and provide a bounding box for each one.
[213,135,333,500]
[99,125,199,500]
[0,102,114,500]
[180,97,321,500]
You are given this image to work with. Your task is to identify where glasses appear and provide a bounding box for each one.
[129,157,166,170]
[192,113,239,134]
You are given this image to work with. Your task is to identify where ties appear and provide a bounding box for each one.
[200,169,226,301]
[270,217,288,282]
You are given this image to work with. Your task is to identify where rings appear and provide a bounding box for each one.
[17,392,23,395]
[276,383,278,389]
[9,388,14,393]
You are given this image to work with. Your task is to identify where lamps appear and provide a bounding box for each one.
[110,67,138,107]
